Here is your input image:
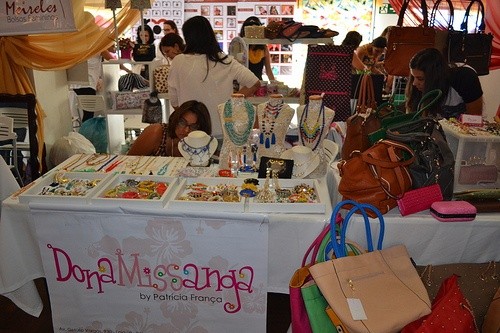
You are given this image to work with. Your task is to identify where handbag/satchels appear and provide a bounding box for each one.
[387,118,453,201]
[397,274,479,333]
[244,20,339,42]
[142,94,164,123]
[429,0,455,63]
[132,43,156,61]
[115,88,150,109]
[368,89,442,161]
[337,139,416,219]
[415,262,500,333]
[78,116,108,153]
[429,201,476,221]
[289,200,431,333]
[341,74,392,162]
[461,0,492,76]
[153,58,171,93]
[383,0,435,76]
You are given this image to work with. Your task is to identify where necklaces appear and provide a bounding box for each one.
[262,100,283,136]
[181,137,213,163]
[298,104,325,153]
[224,100,254,146]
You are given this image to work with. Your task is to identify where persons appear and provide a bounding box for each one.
[132,19,186,81]
[67,12,123,124]
[167,16,260,139]
[405,48,483,120]
[357,36,389,78]
[239,16,276,82]
[341,32,369,74]
[127,99,212,156]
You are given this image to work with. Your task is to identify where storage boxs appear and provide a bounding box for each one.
[439,121,500,193]
[170,177,246,212]
[92,173,179,208]
[18,170,113,205]
[249,178,328,214]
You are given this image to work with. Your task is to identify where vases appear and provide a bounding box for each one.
[121,48,131,59]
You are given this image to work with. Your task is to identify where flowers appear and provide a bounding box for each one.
[105,33,135,49]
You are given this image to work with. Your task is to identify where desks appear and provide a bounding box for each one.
[0,154,336,317]
[324,162,500,324]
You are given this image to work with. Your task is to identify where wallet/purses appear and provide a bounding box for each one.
[456,200,500,212]
[454,189,500,200]
[396,184,443,216]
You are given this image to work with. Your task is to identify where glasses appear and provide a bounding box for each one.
[177,120,195,132]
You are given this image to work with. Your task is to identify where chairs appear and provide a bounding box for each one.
[0,107,30,187]
[77,94,104,116]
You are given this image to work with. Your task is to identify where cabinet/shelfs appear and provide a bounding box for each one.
[95,37,334,132]
